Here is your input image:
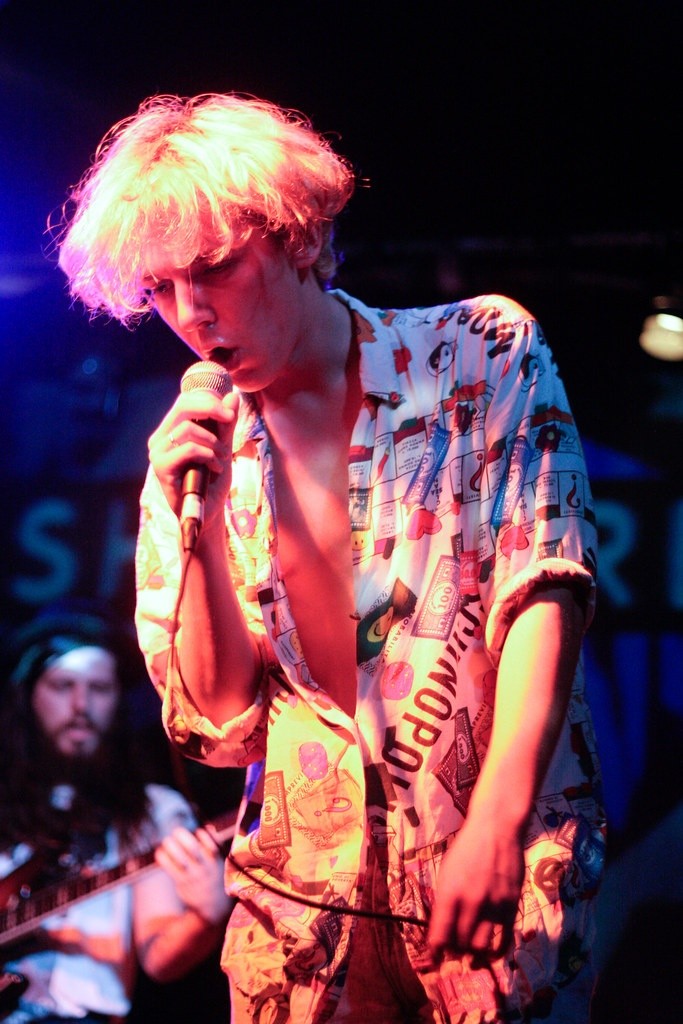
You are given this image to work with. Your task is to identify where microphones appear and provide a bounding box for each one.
[179,361,232,553]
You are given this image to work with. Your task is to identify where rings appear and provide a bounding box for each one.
[168,429,180,450]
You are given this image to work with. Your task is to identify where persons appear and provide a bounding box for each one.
[57,92,604,1024]
[0,617,234,1024]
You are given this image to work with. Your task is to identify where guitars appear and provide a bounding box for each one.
[0,807,251,960]
